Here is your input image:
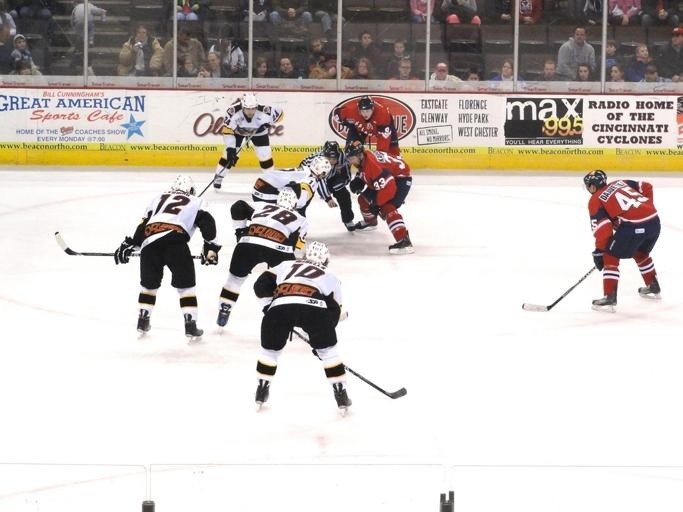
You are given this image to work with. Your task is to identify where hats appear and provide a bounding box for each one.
[672,27,683,35]
[436,62,447,72]
[645,65,657,73]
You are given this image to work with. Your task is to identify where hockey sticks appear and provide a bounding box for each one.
[292,328,406,399]
[54,231,203,259]
[522,263,598,312]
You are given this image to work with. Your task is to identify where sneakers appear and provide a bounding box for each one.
[214,174,224,188]
[342,219,356,232]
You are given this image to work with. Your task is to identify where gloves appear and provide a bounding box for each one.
[200,241,222,265]
[350,176,365,195]
[226,147,238,167]
[369,200,382,216]
[115,236,136,264]
[592,250,604,270]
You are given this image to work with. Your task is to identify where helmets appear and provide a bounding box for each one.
[310,155,332,181]
[345,140,365,157]
[323,141,341,166]
[359,96,373,110]
[243,93,258,109]
[276,186,298,210]
[303,241,331,270]
[584,169,607,190]
[170,173,196,196]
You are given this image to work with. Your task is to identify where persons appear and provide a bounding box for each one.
[346,96,400,157]
[251,156,332,217]
[297,141,356,231]
[344,140,412,250]
[254,241,352,409]
[214,93,285,188]
[114,175,222,337]
[584,170,660,306]
[217,189,308,327]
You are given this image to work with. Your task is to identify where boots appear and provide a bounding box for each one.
[356,218,377,230]
[389,230,413,250]
[217,303,231,328]
[137,310,151,333]
[184,314,203,338]
[638,274,660,295]
[333,383,352,409]
[255,380,269,404]
[592,287,616,306]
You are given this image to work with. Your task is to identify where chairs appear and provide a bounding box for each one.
[0,1,683,81]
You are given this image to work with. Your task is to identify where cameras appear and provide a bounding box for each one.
[135,42,142,49]
